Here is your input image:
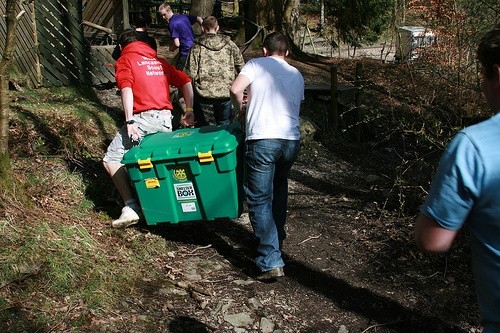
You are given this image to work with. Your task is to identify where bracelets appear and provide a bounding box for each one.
[186,107,194,112]
[125,119,135,124]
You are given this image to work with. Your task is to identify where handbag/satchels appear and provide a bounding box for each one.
[298,116,316,145]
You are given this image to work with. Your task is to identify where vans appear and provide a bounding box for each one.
[395,26,437,60]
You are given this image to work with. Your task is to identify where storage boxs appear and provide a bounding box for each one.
[120,119,243,225]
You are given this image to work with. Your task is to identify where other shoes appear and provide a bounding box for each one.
[112,203,144,229]
[279,240,283,251]
[255,265,285,281]
[169,85,177,93]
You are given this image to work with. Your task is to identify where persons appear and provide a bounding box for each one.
[179,14,248,129]
[413,25,499,333]
[99,28,195,231]
[227,30,306,282]
[158,0,207,112]
